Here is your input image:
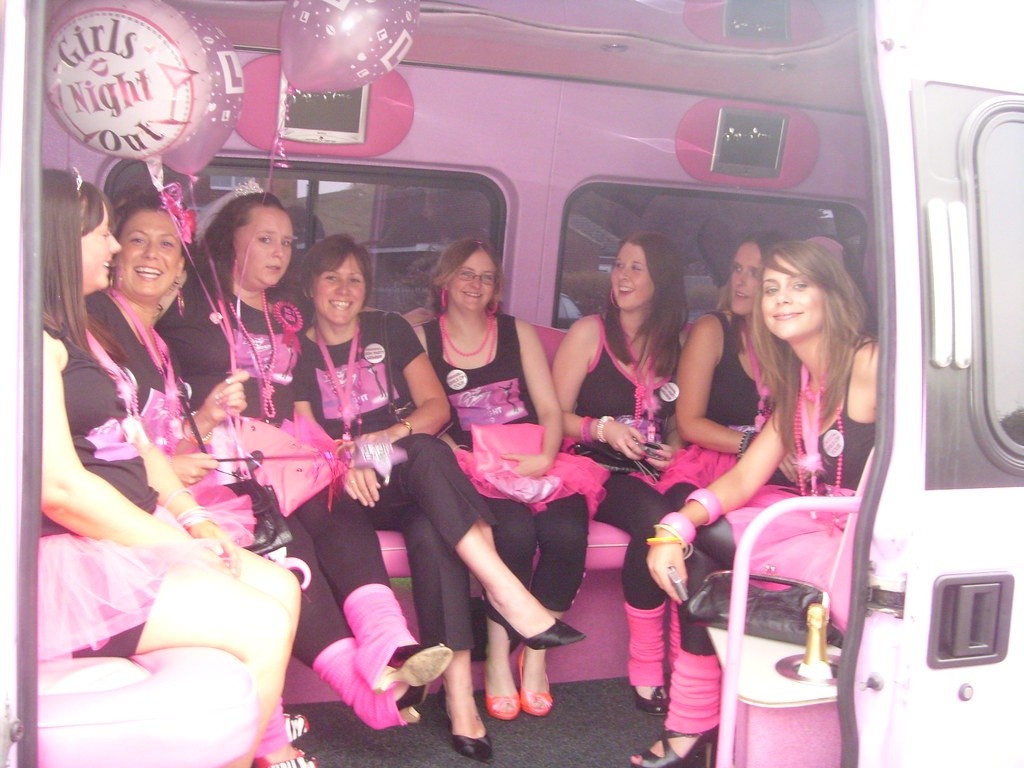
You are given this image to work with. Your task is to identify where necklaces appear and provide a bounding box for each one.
[135,321,183,420]
[796,378,844,497]
[616,344,650,431]
[439,310,497,369]
[104,364,140,422]
[228,292,282,419]
[743,340,773,426]
[326,331,363,441]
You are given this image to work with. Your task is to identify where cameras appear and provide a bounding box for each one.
[635,440,665,460]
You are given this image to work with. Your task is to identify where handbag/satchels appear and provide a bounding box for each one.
[238,416,350,517]
[471,423,546,476]
[213,468,296,556]
[683,568,844,679]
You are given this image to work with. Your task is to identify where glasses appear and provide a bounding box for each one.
[454,270,497,286]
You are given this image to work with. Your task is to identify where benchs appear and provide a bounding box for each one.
[282,493,855,768]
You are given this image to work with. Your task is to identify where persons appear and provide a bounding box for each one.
[292,232,592,763]
[39,170,449,768]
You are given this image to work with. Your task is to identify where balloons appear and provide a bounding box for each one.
[277,0,419,92]
[41,0,245,195]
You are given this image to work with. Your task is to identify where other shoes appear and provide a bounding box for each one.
[252,745,319,768]
[283,712,311,741]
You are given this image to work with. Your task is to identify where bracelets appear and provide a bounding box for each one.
[165,489,194,511]
[178,507,208,529]
[181,411,214,443]
[581,416,594,445]
[737,431,759,457]
[557,231,877,768]
[661,512,696,547]
[597,415,614,444]
[684,490,724,527]
[643,524,692,560]
[400,418,414,435]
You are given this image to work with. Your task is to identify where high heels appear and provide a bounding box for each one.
[450,707,493,766]
[484,659,521,720]
[517,643,554,717]
[395,674,431,724]
[630,726,719,768]
[482,586,585,649]
[374,642,454,695]
[633,685,670,715]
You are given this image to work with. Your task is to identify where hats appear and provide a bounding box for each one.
[806,236,844,266]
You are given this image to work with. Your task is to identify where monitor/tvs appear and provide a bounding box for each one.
[722,0,791,40]
[710,107,790,178]
[276,64,370,144]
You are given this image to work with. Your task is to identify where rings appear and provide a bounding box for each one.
[349,480,355,485]
